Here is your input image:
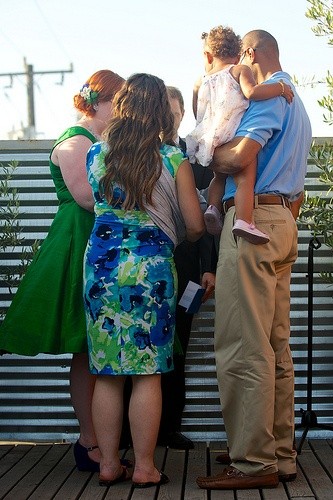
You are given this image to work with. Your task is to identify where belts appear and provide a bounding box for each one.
[223,195,291,214]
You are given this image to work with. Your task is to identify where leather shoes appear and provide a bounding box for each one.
[196,466,278,489]
[216,454,297,482]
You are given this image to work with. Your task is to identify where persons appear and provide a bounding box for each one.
[186,25,294,246]
[195,30,311,488]
[83,72,206,488]
[116,87,218,451]
[0,69,132,472]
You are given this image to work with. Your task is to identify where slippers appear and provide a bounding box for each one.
[98,468,131,486]
[133,471,168,487]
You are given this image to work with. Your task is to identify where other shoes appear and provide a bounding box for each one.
[159,431,194,449]
[232,220,270,245]
[74,440,132,471]
[203,205,222,235]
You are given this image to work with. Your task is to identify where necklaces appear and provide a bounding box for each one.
[85,121,102,141]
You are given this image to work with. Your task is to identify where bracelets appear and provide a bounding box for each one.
[279,82,285,96]
